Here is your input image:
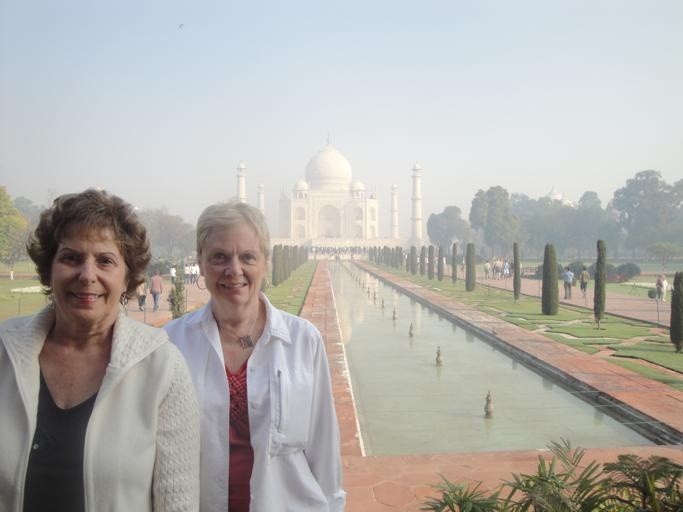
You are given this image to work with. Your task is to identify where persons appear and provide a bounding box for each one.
[169,263,202,285]
[561,268,574,300]
[656,275,668,302]
[1,188,201,512]
[578,265,590,298]
[163,203,347,511]
[151,271,163,310]
[136,281,147,309]
[484,258,514,279]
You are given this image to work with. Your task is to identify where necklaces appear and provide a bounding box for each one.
[224,312,259,350]
